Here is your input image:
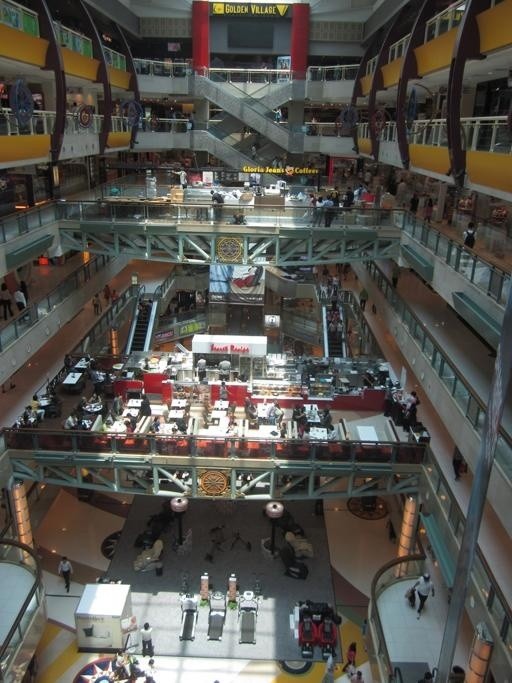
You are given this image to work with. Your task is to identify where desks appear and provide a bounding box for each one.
[62,357,92,394]
[81,397,380,445]
